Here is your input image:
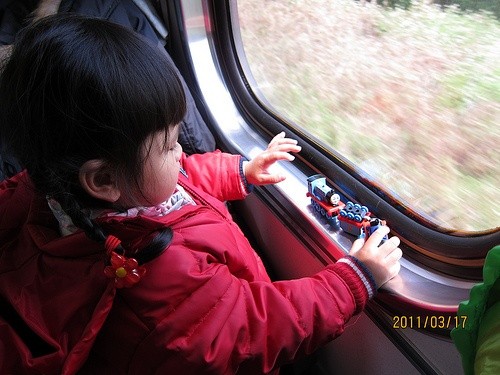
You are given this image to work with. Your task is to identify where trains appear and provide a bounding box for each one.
[305,173,389,248]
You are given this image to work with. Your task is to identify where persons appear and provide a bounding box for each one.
[1,13,405,374]
